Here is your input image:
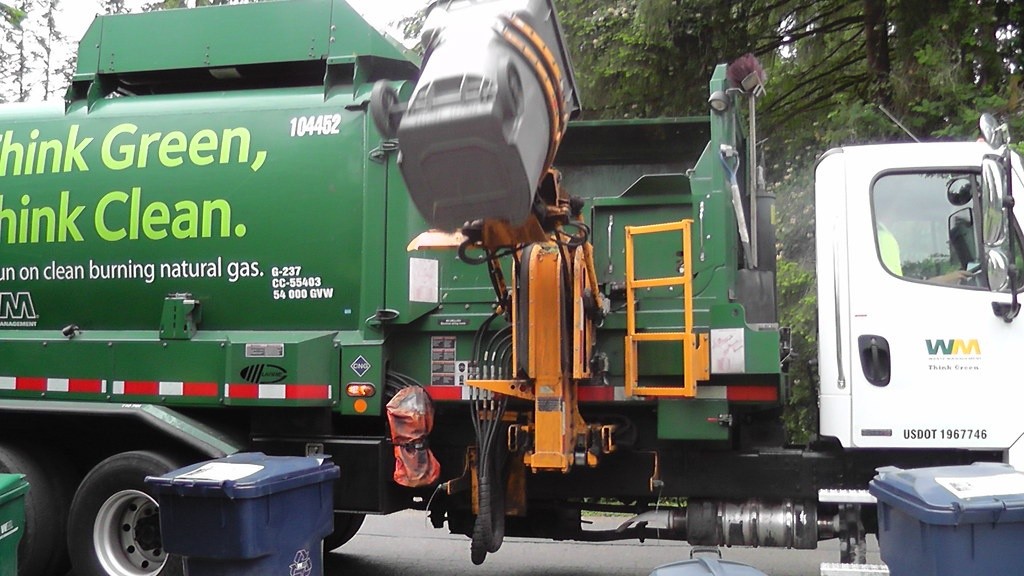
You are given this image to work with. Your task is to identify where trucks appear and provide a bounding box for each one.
[1,0,1023,576]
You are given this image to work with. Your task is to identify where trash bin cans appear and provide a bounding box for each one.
[0,472,31,576]
[142,451,339,576]
[371,1,582,234]
[869,461,1024,576]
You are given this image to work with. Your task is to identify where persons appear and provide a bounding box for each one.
[873,182,975,283]
